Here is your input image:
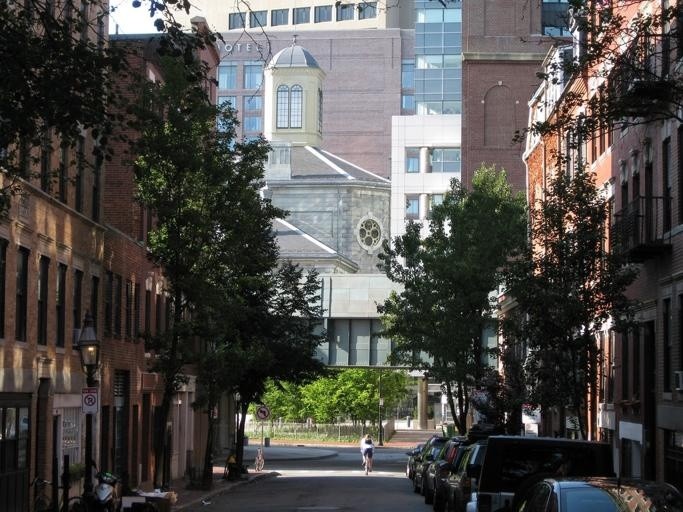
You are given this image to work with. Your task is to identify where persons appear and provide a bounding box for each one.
[360,434,374,472]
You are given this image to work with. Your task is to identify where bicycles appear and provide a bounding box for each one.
[224,447,236,481]
[254,448,265,471]
[29,477,92,512]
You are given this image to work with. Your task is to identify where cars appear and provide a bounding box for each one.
[406,435,683,512]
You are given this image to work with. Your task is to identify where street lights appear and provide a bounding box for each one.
[377,395,384,445]
[76,308,102,512]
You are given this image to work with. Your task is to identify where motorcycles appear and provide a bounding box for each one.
[91,458,129,512]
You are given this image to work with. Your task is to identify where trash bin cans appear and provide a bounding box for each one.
[265,438,270,446]
[442,424,455,438]
